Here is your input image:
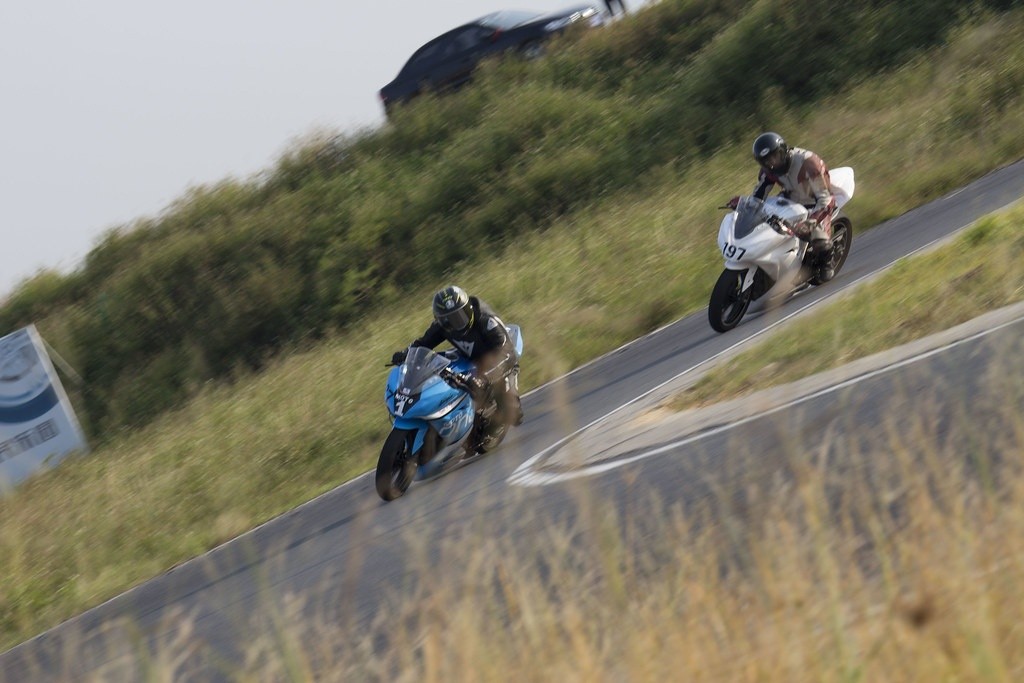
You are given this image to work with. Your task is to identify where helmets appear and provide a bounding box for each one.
[753,133,788,173]
[432,286,475,336]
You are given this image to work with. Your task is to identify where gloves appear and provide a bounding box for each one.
[391,349,406,366]
[727,196,740,211]
[793,217,817,236]
[464,375,487,396]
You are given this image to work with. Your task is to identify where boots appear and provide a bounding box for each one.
[823,245,836,279]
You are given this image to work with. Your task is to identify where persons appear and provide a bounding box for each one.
[727,133,834,282]
[392,285,524,447]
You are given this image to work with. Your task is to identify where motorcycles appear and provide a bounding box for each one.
[375,322,523,501]
[707,165,855,334]
